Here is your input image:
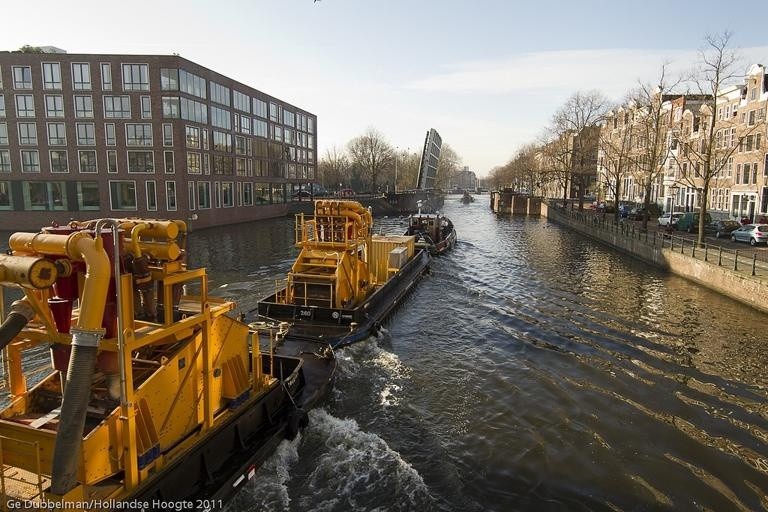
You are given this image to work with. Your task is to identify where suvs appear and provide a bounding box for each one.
[674,212,712,233]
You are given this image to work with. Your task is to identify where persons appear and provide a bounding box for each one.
[731,215,767,226]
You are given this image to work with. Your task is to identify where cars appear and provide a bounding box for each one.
[292,189,355,200]
[657,212,685,228]
[617,204,633,218]
[729,223,768,247]
[596,203,615,214]
[627,208,650,222]
[705,219,742,238]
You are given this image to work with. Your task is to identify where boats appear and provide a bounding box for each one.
[398,200,457,257]
[1,213,340,511]
[245,196,436,347]
[459,190,474,204]
